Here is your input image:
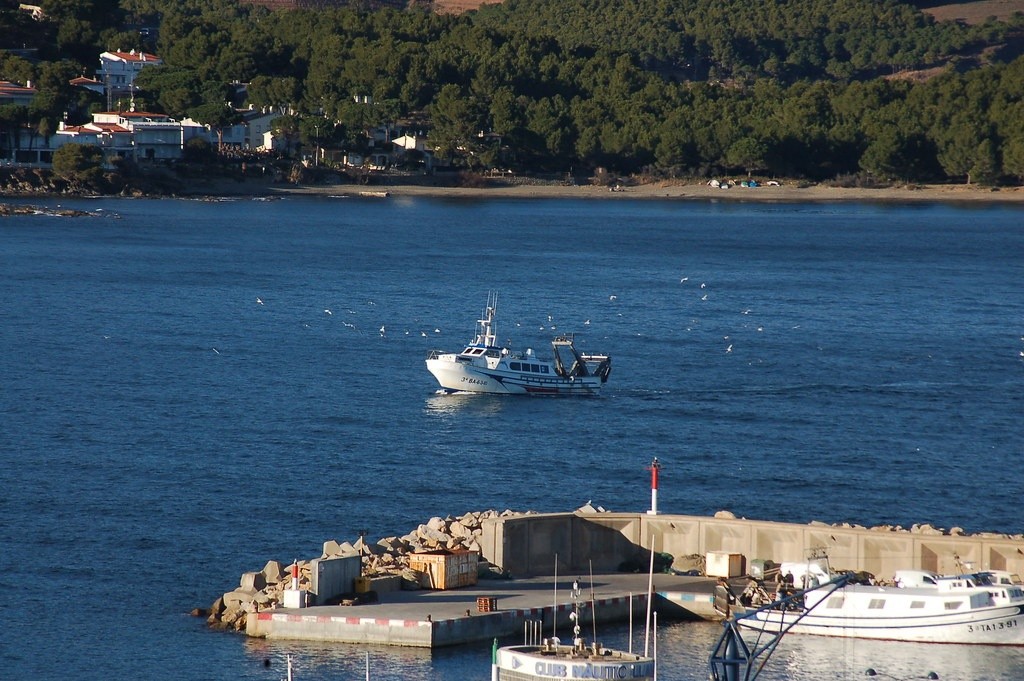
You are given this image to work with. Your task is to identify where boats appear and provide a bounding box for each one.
[493,535,874,681]
[712,552,1024,646]
[425,290,612,395]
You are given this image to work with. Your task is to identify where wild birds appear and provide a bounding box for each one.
[211,275,824,366]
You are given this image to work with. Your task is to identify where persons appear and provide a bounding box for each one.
[740,570,798,610]
